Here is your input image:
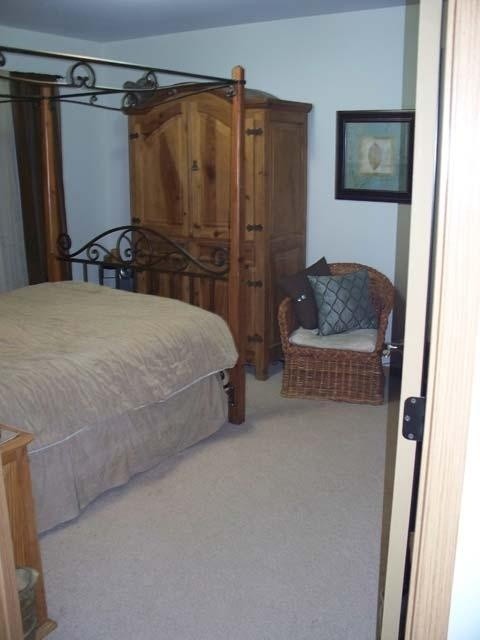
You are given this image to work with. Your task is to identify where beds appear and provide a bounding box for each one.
[1,215,249,538]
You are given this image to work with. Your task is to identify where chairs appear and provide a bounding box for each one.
[277,262,395,406]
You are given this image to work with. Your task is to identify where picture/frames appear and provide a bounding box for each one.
[334,107,414,205]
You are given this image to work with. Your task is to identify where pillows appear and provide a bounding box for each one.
[278,255,380,337]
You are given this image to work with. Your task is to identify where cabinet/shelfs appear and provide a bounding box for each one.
[127,82,313,381]
